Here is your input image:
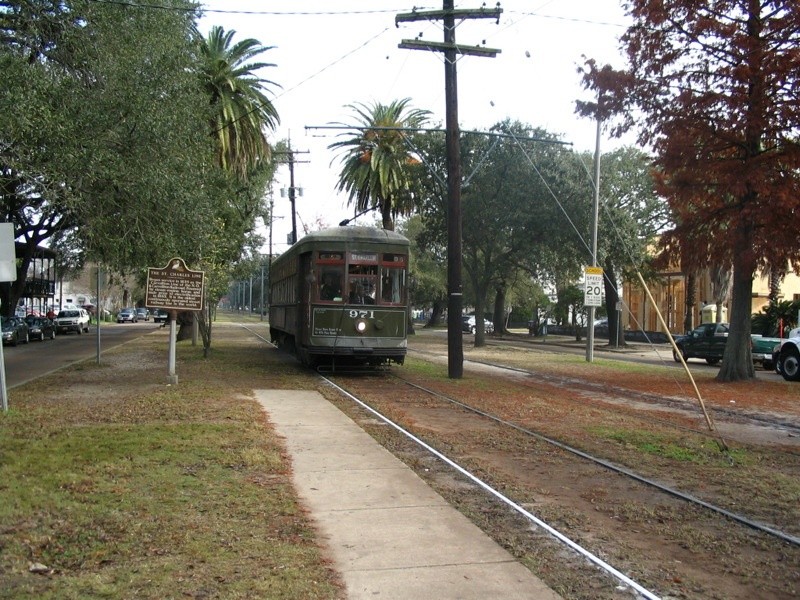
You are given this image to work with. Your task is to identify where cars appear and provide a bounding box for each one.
[23,317,57,341]
[462,315,493,335]
[1,315,31,346]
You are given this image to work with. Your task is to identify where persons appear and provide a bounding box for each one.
[698,300,708,325]
[321,276,337,300]
[46,308,55,322]
[351,285,375,305]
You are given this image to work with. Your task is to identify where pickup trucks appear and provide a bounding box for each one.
[673,323,781,366]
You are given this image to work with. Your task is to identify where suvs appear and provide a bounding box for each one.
[53,308,90,334]
[135,308,150,321]
[117,308,136,323]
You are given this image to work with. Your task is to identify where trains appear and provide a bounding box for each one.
[267,224,412,371]
